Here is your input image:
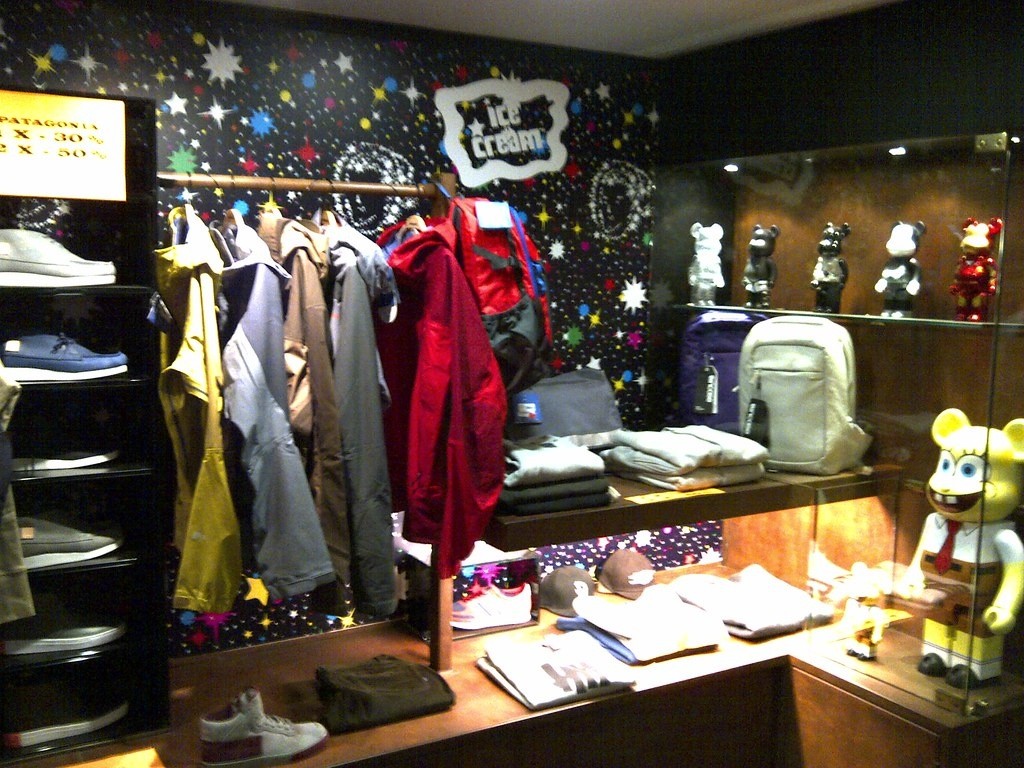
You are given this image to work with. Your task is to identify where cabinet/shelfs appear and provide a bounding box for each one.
[767,664,1024,768]
[0,262,168,768]
[484,297,1014,713]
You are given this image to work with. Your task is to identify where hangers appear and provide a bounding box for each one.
[165,166,458,275]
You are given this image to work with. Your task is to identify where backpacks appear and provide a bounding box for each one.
[738,314,873,474]
[679,309,768,434]
[428,178,553,394]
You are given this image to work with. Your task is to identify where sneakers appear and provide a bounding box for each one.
[1,681,129,748]
[17,508,123,570]
[196,686,330,768]
[0,332,128,381]
[11,435,119,472]
[0,228,116,287]
[450,582,532,629]
[0,591,125,654]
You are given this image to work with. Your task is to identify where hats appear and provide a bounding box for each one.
[539,564,594,617]
[598,549,658,600]
